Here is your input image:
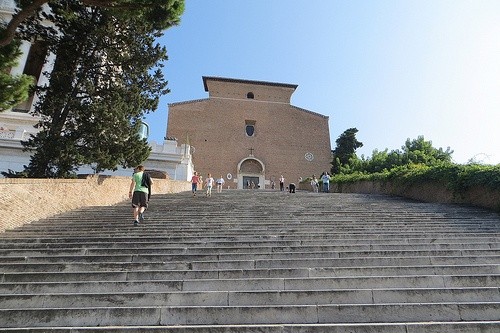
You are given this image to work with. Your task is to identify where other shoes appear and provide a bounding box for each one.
[140,215,144,220]
[134,221,138,226]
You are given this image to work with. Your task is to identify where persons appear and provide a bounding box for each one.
[215,175,225,193]
[191,172,201,196]
[286,183,296,193]
[272,181,276,189]
[279,175,285,191]
[204,173,214,197]
[129,165,154,224]
[311,174,320,193]
[321,172,332,193]
